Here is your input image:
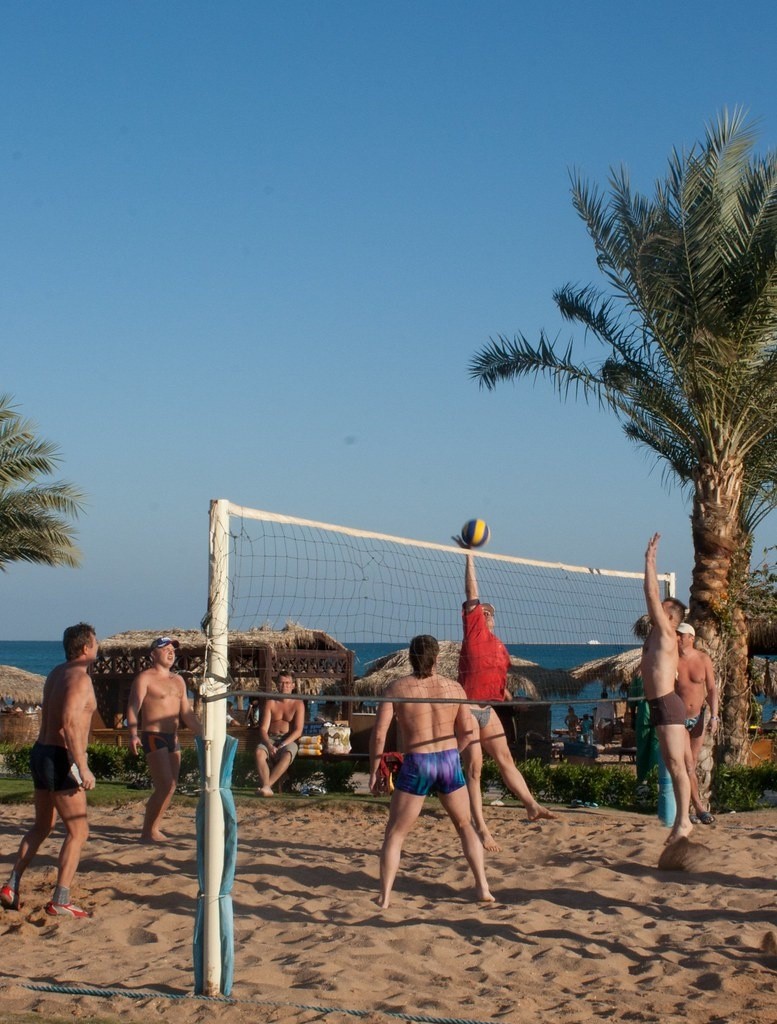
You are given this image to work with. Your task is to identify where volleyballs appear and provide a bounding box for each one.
[460,518,491,548]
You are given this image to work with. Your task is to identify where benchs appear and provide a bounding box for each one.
[273,752,404,793]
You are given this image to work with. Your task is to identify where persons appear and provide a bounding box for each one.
[227,696,260,727]
[0,700,42,714]
[564,690,617,746]
[449,531,560,852]
[253,671,304,797]
[634,614,719,829]
[368,635,496,908]
[769,711,777,722]
[125,637,203,843]
[642,532,694,845]
[0,623,100,919]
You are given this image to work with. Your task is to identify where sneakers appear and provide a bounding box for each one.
[0,886,20,911]
[46,899,90,917]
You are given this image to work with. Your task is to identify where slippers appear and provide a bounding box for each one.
[700,813,716,825]
[689,815,701,824]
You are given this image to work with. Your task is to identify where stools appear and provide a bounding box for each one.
[615,746,637,762]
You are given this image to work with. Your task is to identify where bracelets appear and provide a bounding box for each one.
[711,716,718,721]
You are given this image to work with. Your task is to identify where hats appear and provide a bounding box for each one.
[676,623,695,636]
[151,636,180,650]
[480,603,495,615]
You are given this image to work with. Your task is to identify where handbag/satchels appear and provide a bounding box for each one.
[321,721,351,755]
